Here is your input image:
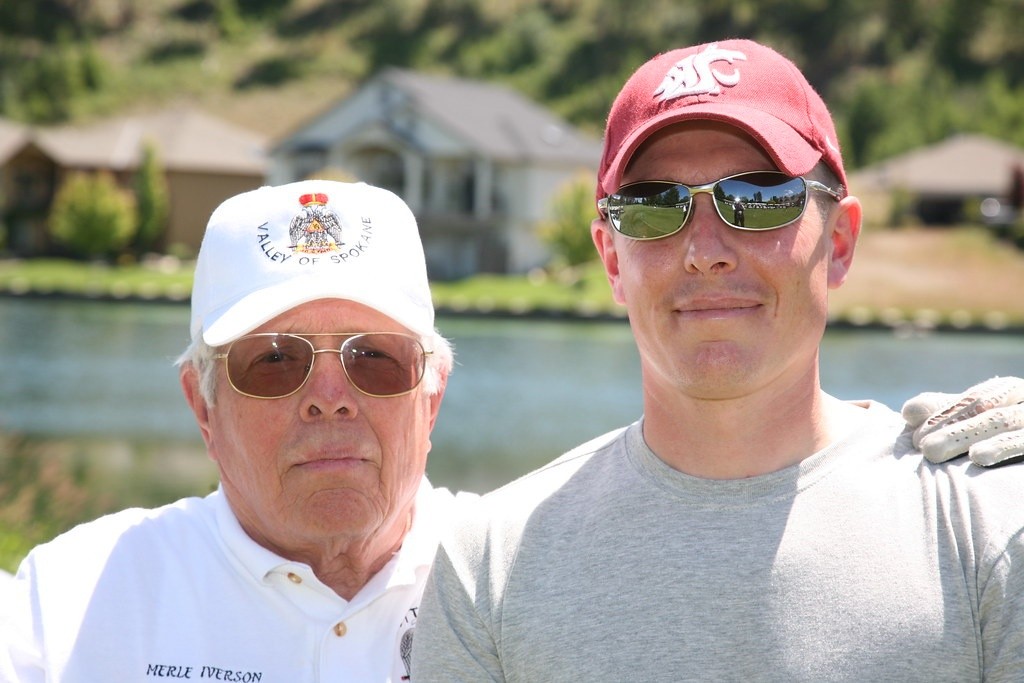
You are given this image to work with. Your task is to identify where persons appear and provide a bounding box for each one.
[410,38,1024,683]
[0,181,1024,683]
[732,200,745,227]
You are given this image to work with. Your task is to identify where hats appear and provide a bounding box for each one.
[190,179,436,347]
[595,39,850,220]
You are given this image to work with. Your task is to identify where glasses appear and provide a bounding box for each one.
[212,332,436,399]
[597,169,841,240]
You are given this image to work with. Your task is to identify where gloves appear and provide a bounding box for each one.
[901,376,1024,467]
